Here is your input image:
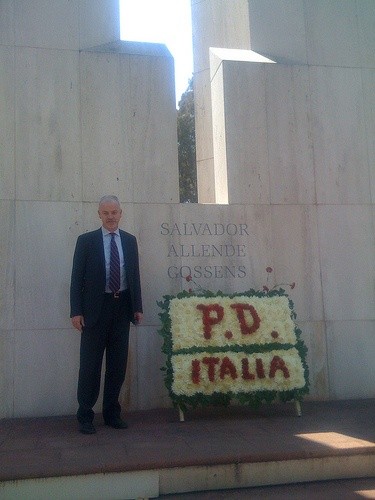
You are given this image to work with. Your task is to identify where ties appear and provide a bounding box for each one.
[109,232,121,293]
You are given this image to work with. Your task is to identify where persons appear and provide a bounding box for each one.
[70,194,145,434]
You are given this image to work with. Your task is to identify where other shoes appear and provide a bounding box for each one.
[105,418,128,428]
[80,422,96,434]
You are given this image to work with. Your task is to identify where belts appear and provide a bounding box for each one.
[106,291,130,302]
